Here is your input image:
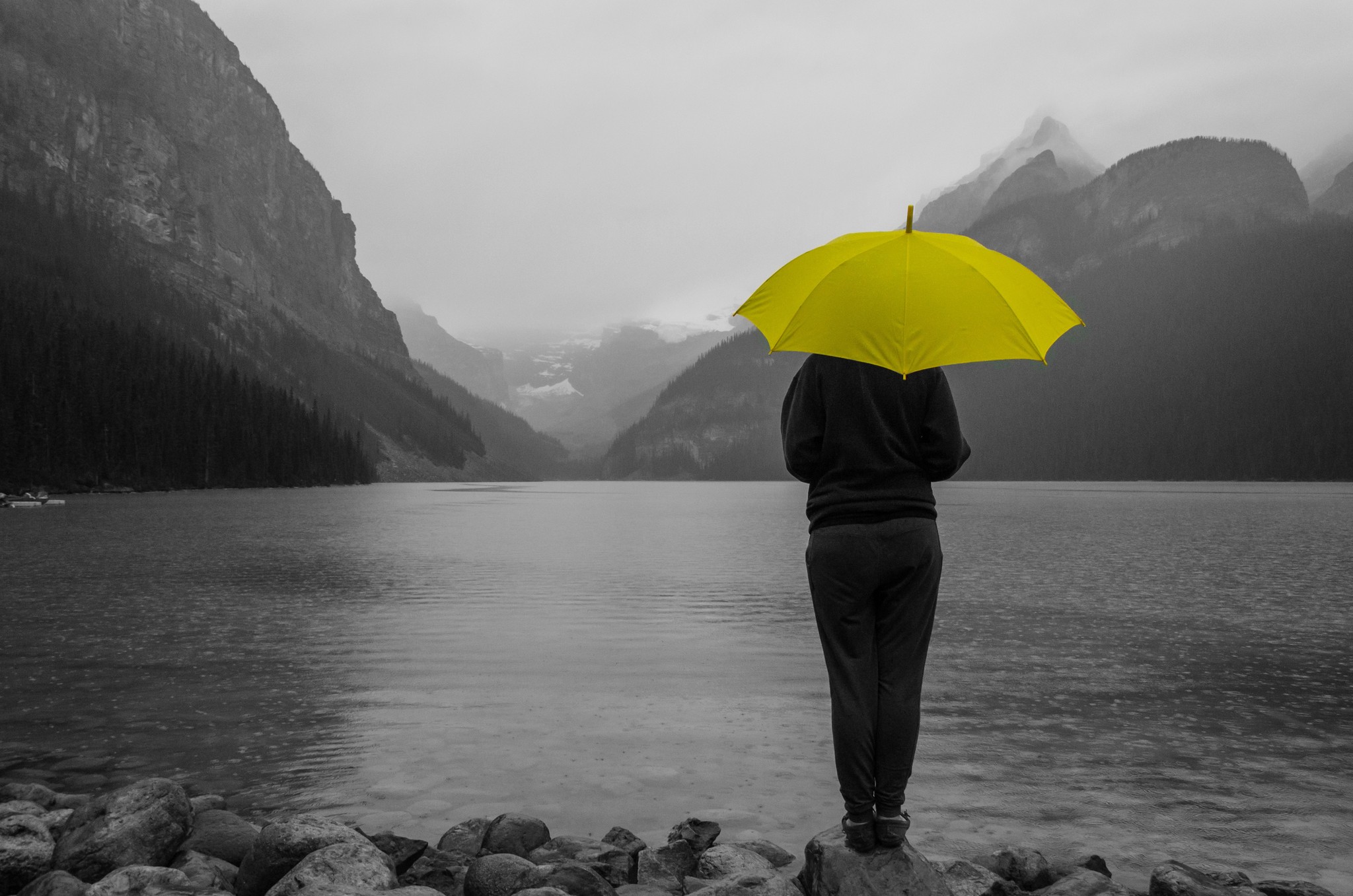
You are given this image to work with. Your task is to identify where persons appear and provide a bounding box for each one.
[781,351,977,853]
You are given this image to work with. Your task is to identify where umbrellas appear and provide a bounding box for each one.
[727,202,1088,378]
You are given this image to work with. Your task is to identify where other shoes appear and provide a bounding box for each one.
[873,812,910,846]
[841,811,877,851]
